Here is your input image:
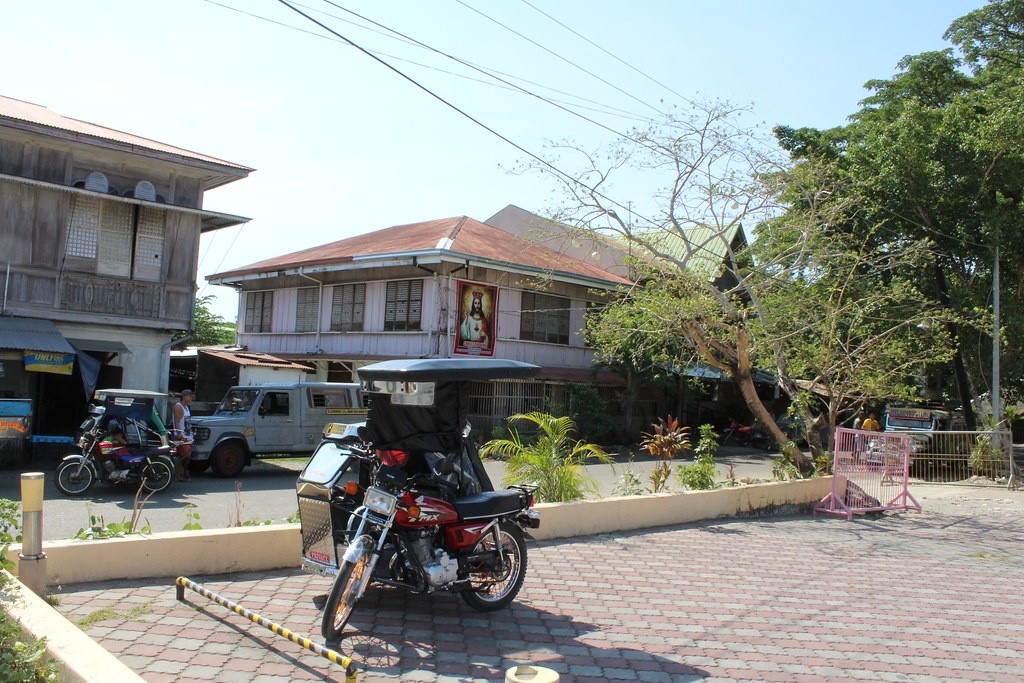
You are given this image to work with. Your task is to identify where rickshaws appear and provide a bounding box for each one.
[296,358,544,641]
[716,399,786,448]
[54,388,185,497]
[0,398,35,470]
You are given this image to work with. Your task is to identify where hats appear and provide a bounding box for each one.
[181,389,193,397]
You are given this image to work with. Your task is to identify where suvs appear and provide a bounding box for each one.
[182,382,369,479]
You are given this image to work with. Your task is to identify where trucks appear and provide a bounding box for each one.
[866,406,979,477]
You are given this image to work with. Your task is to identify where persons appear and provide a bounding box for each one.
[172,389,193,482]
[854,411,881,462]
[100,428,127,471]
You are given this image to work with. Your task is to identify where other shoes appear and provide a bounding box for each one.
[185,475,191,481]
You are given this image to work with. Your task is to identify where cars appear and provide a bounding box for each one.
[775,413,829,448]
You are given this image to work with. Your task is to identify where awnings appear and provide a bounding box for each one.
[0,316,130,354]
